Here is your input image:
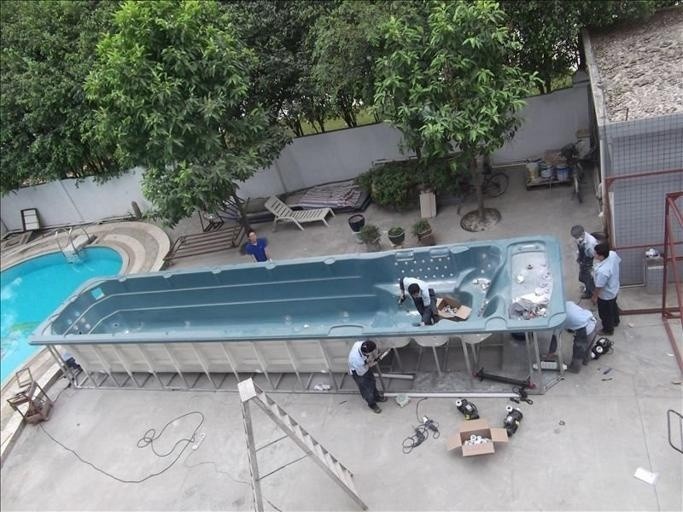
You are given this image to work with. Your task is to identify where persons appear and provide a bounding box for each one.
[591,243,622,336]
[399,275,437,327]
[570,225,598,300]
[244,229,272,263]
[546,301,598,371]
[347,340,387,414]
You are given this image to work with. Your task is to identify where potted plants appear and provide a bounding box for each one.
[387,226,406,245]
[359,224,383,252]
[412,219,436,245]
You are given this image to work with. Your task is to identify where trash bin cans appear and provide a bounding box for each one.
[420,191,437,218]
[643,251,668,294]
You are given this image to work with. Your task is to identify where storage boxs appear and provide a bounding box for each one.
[448,417,510,458]
[433,294,474,321]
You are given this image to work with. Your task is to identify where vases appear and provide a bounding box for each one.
[348,214,365,232]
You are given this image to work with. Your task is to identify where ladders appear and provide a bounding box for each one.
[237,378,370,512]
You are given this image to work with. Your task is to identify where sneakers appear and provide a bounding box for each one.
[376,396,387,402]
[374,406,381,413]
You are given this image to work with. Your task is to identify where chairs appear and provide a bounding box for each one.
[264,194,336,231]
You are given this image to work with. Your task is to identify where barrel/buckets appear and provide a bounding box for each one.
[540,160,553,178]
[555,163,569,182]
